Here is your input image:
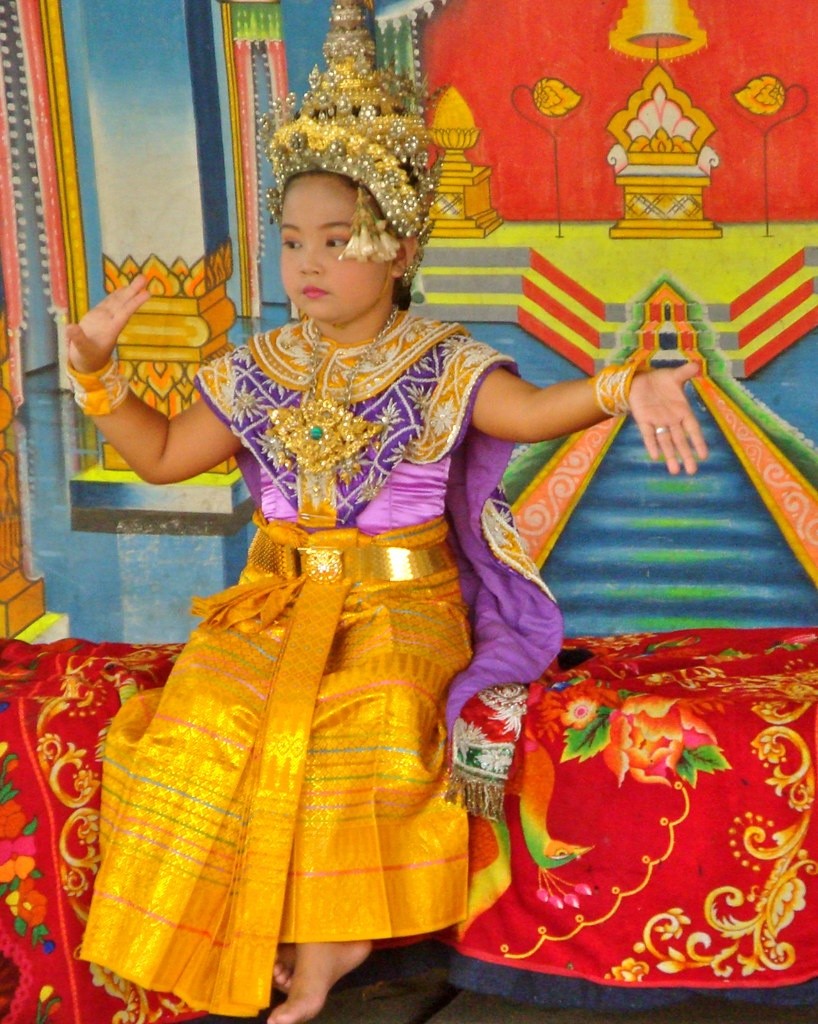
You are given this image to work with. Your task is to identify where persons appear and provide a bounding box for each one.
[66,1,710,1024]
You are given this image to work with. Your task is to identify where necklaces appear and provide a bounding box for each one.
[265,303,398,472]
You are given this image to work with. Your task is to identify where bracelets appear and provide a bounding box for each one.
[588,360,642,416]
[66,358,129,417]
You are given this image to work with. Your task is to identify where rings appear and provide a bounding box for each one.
[656,427,669,435]
